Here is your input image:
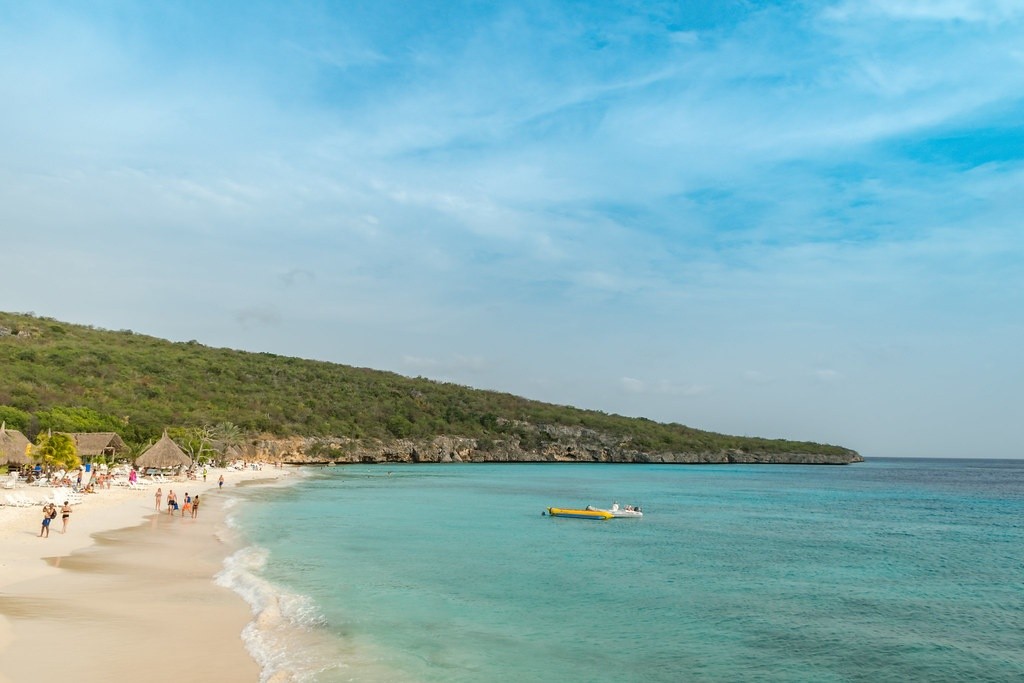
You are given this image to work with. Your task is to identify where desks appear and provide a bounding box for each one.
[28,470,41,479]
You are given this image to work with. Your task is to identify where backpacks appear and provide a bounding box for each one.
[50,509,57,519]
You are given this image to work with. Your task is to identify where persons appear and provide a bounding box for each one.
[99,473,104,489]
[155,488,162,510]
[29,463,42,470]
[76,469,83,488]
[52,477,58,485]
[107,471,112,489]
[66,476,70,485]
[129,468,137,482]
[191,495,200,518]
[203,468,208,482]
[59,501,72,534]
[217,475,224,488]
[167,490,177,515]
[182,492,191,516]
[37,503,54,538]
[90,469,96,491]
[612,500,618,511]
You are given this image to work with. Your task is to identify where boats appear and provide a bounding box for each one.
[586,506,643,518]
[547,506,615,520]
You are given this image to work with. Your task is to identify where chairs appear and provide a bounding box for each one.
[114,474,173,490]
[0,476,86,509]
